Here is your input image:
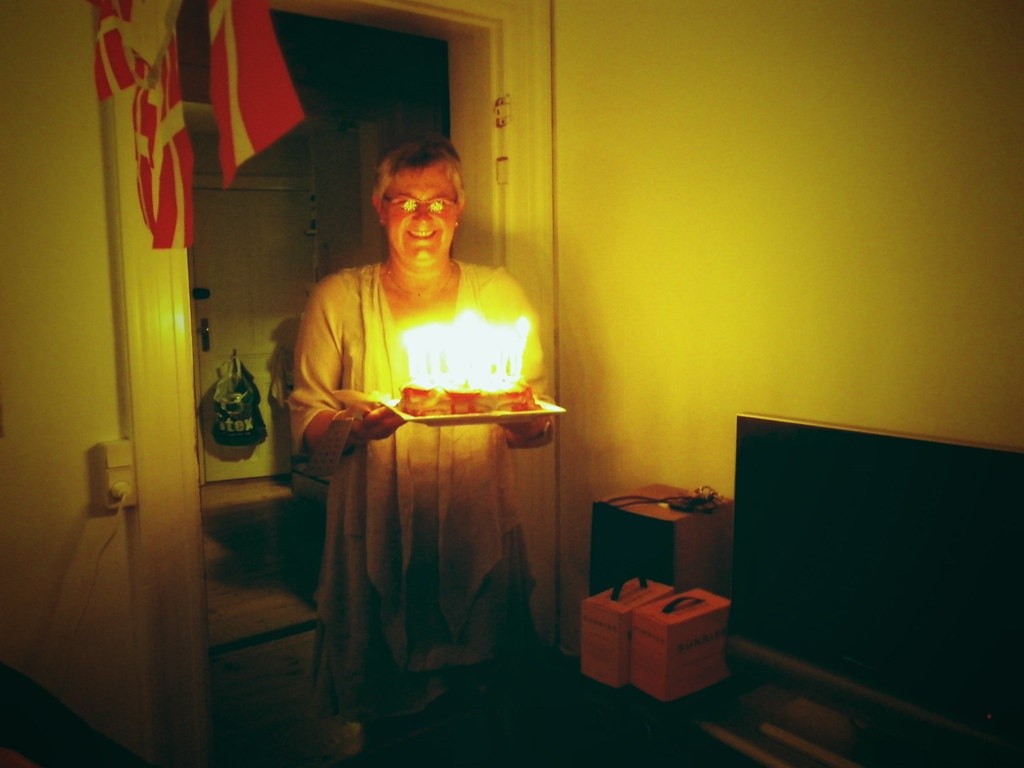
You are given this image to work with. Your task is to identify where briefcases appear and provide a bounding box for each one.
[628,587,731,702]
[580,570,674,688]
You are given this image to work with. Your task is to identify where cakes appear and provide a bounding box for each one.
[398,378,541,417]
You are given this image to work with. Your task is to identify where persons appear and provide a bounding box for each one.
[287,129,558,768]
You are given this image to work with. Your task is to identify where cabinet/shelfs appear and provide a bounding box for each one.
[177,101,326,512]
[586,481,734,596]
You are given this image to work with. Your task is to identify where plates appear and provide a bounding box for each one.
[378,398,567,426]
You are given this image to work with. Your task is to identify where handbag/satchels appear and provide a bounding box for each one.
[211,354,268,447]
[271,350,297,411]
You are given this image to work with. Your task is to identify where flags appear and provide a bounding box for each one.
[206,0,305,191]
[87,0,195,251]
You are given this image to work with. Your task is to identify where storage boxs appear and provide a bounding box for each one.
[579,573,733,694]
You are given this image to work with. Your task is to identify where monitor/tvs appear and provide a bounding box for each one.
[726,410,1024,768]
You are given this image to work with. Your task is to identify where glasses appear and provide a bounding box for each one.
[381,193,458,217]
